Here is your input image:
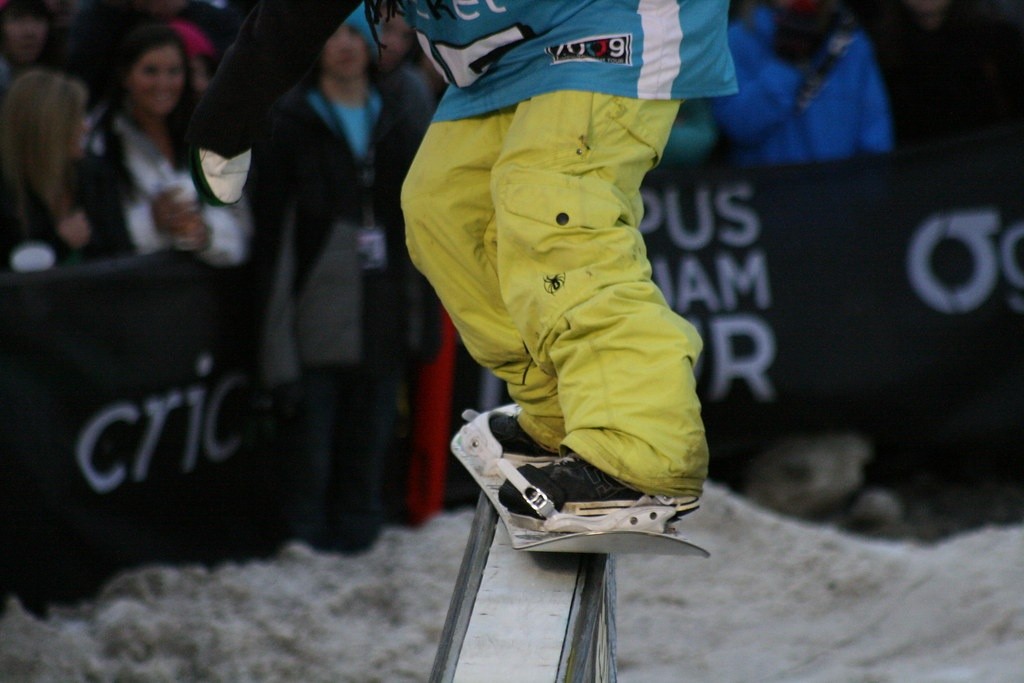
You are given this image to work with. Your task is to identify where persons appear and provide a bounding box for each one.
[247,0,427,565]
[178,0,740,522]
[0,0,1024,291]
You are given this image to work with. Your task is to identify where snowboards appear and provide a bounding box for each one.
[452,402,712,560]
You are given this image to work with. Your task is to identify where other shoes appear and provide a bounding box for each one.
[540,447,701,516]
[490,410,560,456]
